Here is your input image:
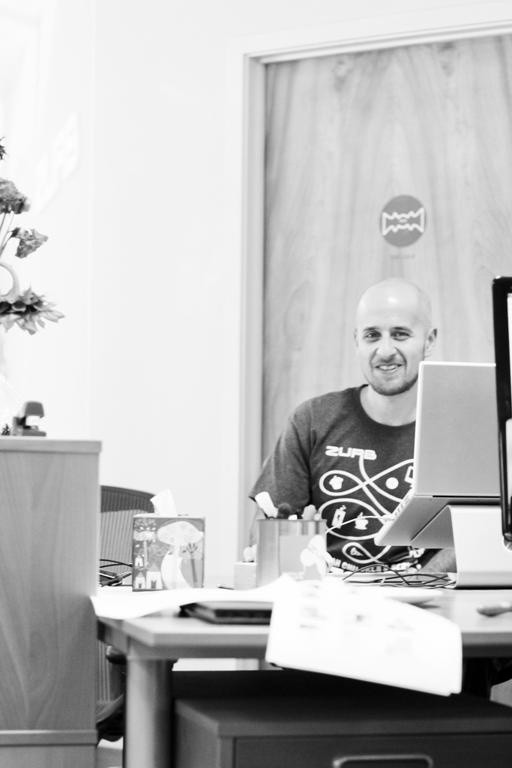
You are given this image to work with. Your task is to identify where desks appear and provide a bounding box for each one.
[92,586,512,768]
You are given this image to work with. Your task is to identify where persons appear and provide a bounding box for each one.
[246,277,459,581]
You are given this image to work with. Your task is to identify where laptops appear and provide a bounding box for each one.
[373,361,502,546]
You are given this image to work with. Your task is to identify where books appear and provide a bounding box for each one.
[182,598,270,623]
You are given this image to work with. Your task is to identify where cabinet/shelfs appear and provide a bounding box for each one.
[169,694,512,768]
[1,431,105,768]
